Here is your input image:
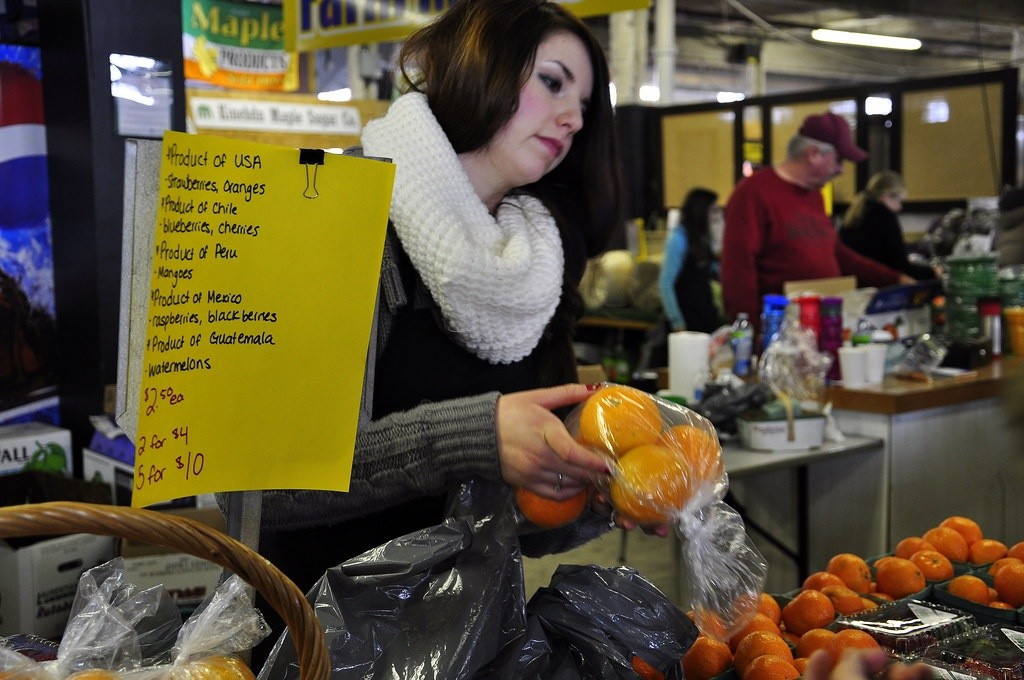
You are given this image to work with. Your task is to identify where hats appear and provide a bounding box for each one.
[799,112,868,161]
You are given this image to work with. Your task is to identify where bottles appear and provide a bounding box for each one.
[978,301,1003,358]
[761,294,843,380]
[730,313,755,376]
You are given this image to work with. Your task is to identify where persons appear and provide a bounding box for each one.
[839,171,941,282]
[722,114,913,338]
[658,187,722,334]
[214,0,614,592]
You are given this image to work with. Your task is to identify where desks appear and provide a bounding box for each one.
[669,428,883,605]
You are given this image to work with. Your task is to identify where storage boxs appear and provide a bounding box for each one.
[861,283,932,344]
[0,533,115,640]
[733,416,827,452]
[83,448,217,510]
[0,421,73,478]
[115,508,229,623]
[771,551,1024,680]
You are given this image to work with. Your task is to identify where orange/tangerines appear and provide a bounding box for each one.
[516,383,722,529]
[626,517,1024,680]
[0,656,256,680]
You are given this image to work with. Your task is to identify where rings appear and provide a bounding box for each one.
[558,474,561,488]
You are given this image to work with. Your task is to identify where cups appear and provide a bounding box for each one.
[666,330,712,406]
[1008,308,1024,354]
[836,342,888,389]
